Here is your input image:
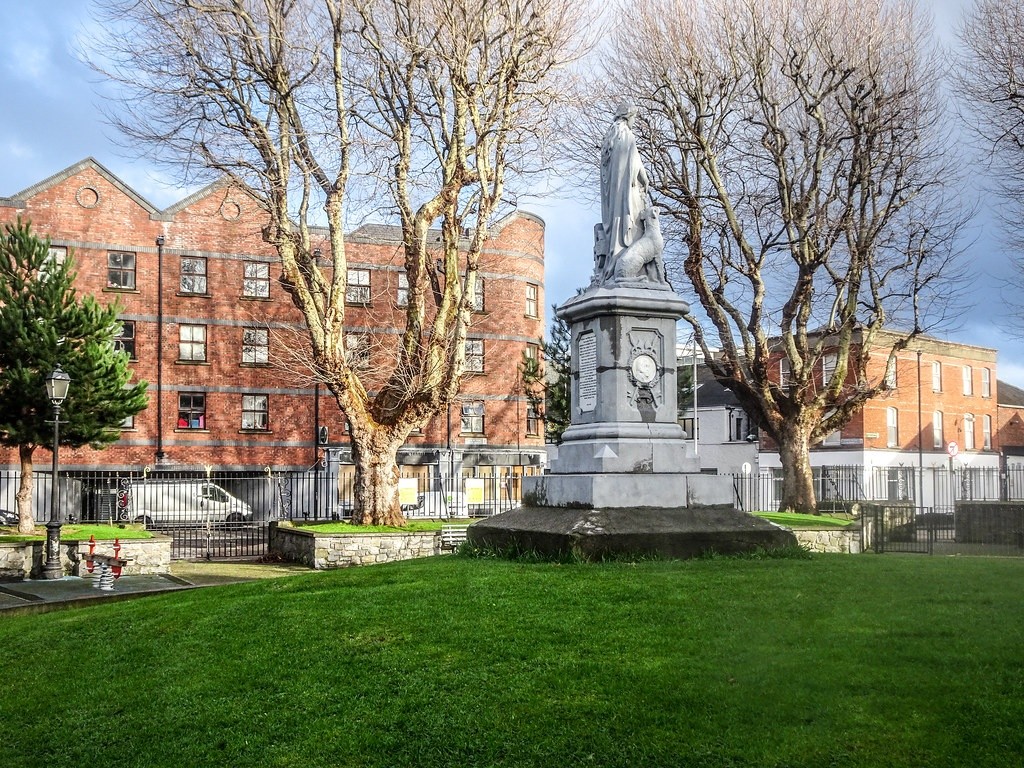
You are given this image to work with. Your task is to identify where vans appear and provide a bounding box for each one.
[126,478,254,532]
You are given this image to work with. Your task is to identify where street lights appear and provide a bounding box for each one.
[39,362,74,581]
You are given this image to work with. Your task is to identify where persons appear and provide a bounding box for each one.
[599,101,666,285]
